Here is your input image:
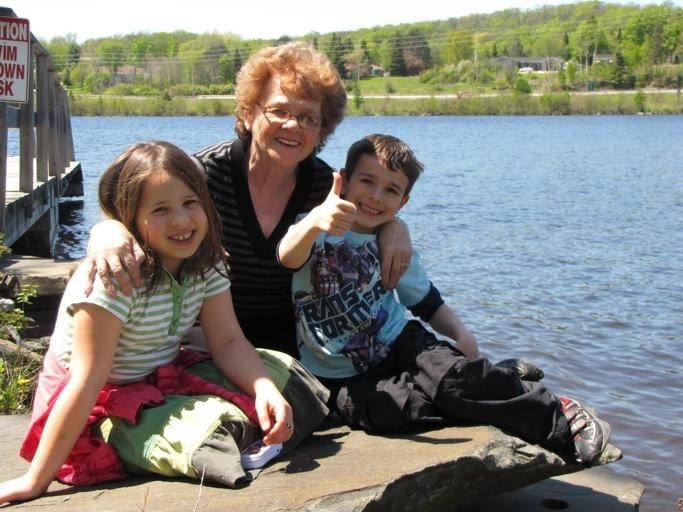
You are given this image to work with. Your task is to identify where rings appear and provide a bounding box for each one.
[400,260,408,267]
[285,421,292,429]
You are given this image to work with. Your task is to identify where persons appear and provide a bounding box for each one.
[0,139,332,505]
[271,130,615,465]
[79,38,417,362]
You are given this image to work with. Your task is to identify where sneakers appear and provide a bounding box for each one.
[555,397,603,467]
[496,359,544,382]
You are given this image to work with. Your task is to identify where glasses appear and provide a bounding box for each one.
[253,100,325,130]
